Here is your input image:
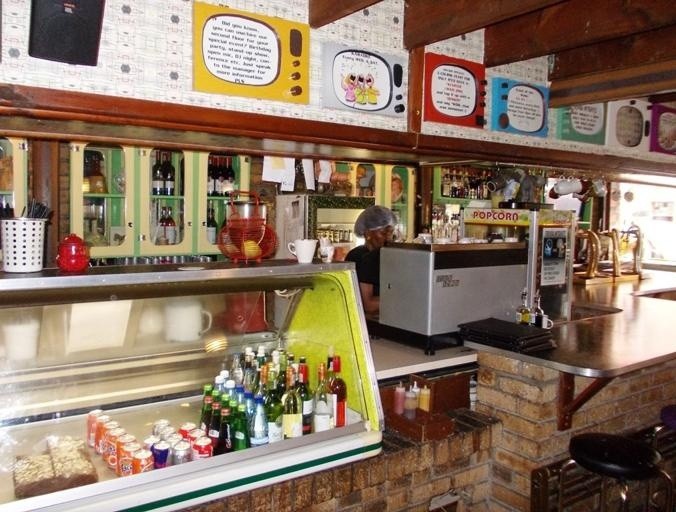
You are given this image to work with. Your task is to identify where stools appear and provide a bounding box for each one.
[650,402,675,456]
[557,432,674,510]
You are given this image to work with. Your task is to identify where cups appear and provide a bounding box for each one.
[487,169,608,203]
[542,314,553,330]
[287,238,334,264]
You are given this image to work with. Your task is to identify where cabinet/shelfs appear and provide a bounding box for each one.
[0,257,386,512]
[0,135,418,264]
[433,168,594,237]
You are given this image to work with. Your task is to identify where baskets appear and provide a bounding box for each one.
[218,189,277,265]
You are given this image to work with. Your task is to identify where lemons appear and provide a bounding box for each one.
[241,241,262,258]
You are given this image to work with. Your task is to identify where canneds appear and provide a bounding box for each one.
[87,409,214,477]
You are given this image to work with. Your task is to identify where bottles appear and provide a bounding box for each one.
[516,287,543,330]
[317,228,352,242]
[83,148,236,266]
[200,345,347,455]
[56,233,88,272]
[431,164,488,240]
[394,379,430,419]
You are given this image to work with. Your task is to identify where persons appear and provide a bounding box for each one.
[343,206,397,313]
